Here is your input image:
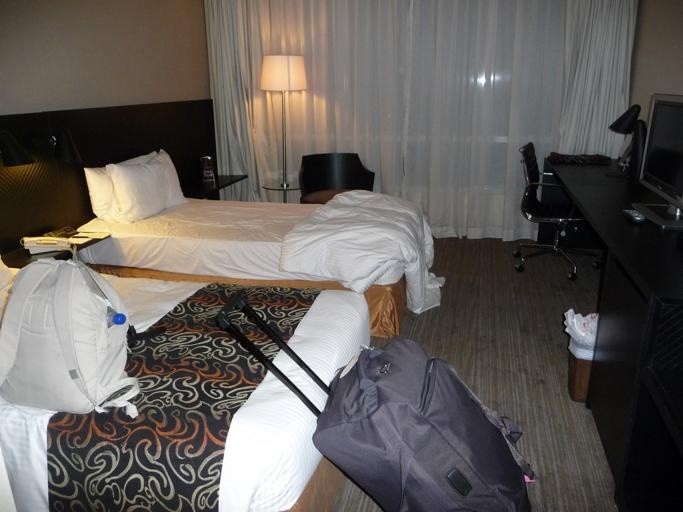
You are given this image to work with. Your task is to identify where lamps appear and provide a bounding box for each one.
[259,53,309,202]
[606,104,647,186]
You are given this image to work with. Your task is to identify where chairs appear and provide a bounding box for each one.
[297,152,375,204]
[512,142,600,280]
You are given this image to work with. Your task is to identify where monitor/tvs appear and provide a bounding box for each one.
[631,93,683,230]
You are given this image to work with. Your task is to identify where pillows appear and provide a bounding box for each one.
[1,260,16,291]
[82,148,190,224]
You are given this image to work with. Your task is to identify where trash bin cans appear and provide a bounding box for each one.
[568,339,595,403]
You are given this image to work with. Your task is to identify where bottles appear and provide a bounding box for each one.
[107,306,128,330]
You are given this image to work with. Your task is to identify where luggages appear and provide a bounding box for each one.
[312,336,530,510]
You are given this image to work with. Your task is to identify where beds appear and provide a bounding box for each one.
[1,265,371,510]
[69,198,446,339]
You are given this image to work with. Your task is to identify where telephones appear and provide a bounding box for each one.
[23,237,71,255]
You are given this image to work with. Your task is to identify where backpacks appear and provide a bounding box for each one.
[0,259,143,419]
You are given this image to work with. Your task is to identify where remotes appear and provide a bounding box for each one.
[623,209,646,223]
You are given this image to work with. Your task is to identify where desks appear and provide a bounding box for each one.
[535,158,683,511]
[0,232,113,270]
